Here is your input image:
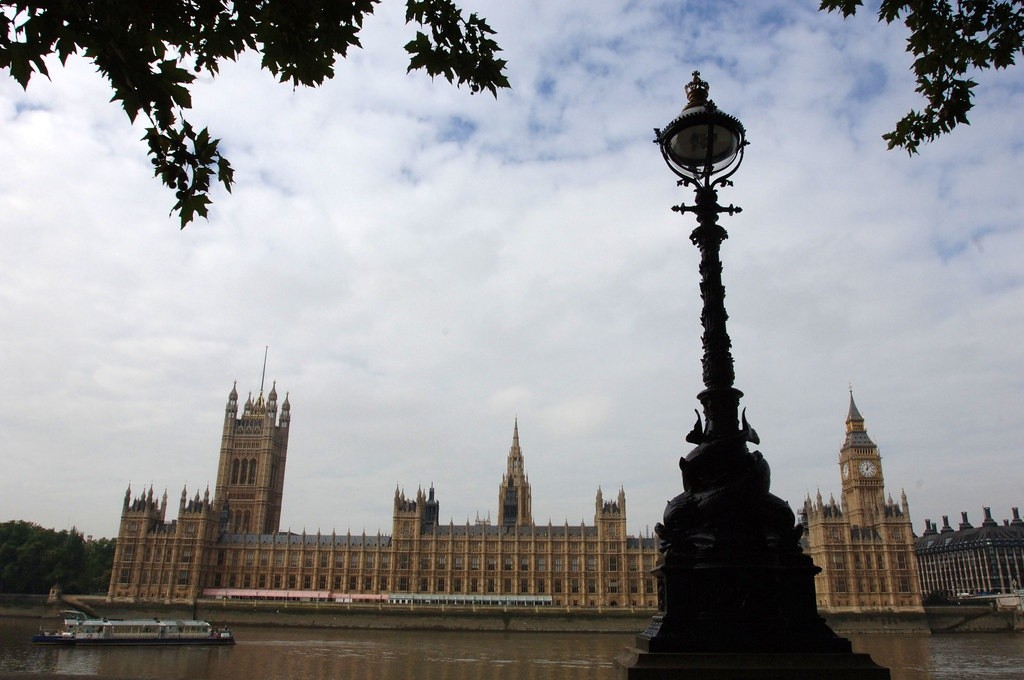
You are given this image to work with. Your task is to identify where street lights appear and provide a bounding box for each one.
[632,69,856,657]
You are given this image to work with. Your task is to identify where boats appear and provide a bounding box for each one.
[25,610,237,647]
[920,588,1024,634]
